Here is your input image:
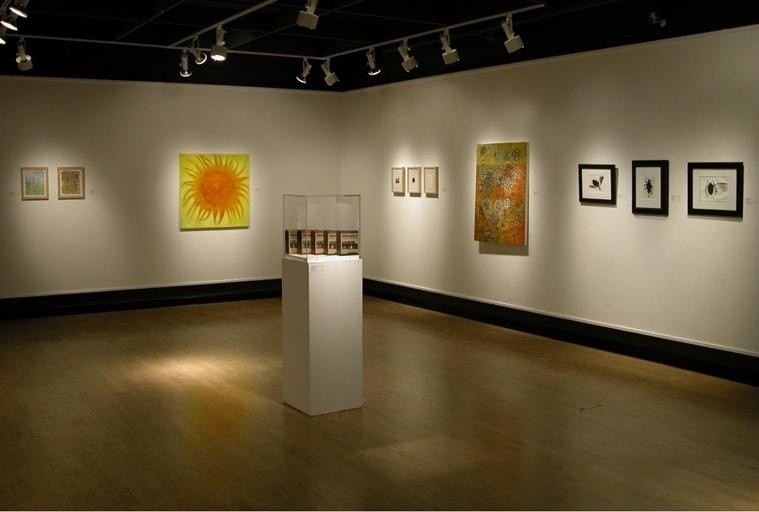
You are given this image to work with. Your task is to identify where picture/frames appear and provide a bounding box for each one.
[631,159,670,215]
[19,167,85,201]
[577,163,616,205]
[686,161,745,218]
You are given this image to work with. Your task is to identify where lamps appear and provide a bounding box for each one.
[14,38,33,72]
[178,23,228,80]
[296,59,314,85]
[320,14,527,88]
[294,0,320,32]
